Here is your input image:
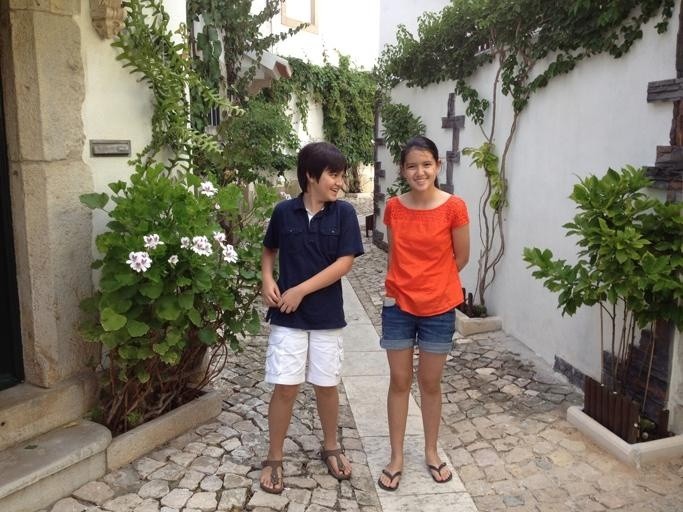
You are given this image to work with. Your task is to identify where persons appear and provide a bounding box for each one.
[257,141,366,496]
[376,135,472,491]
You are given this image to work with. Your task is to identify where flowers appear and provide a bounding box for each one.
[77,158,275,386]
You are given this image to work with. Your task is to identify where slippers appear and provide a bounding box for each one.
[425,459,453,483]
[260,458,284,494]
[377,464,402,492]
[318,440,351,480]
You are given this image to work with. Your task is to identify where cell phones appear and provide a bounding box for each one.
[384,297,396,307]
